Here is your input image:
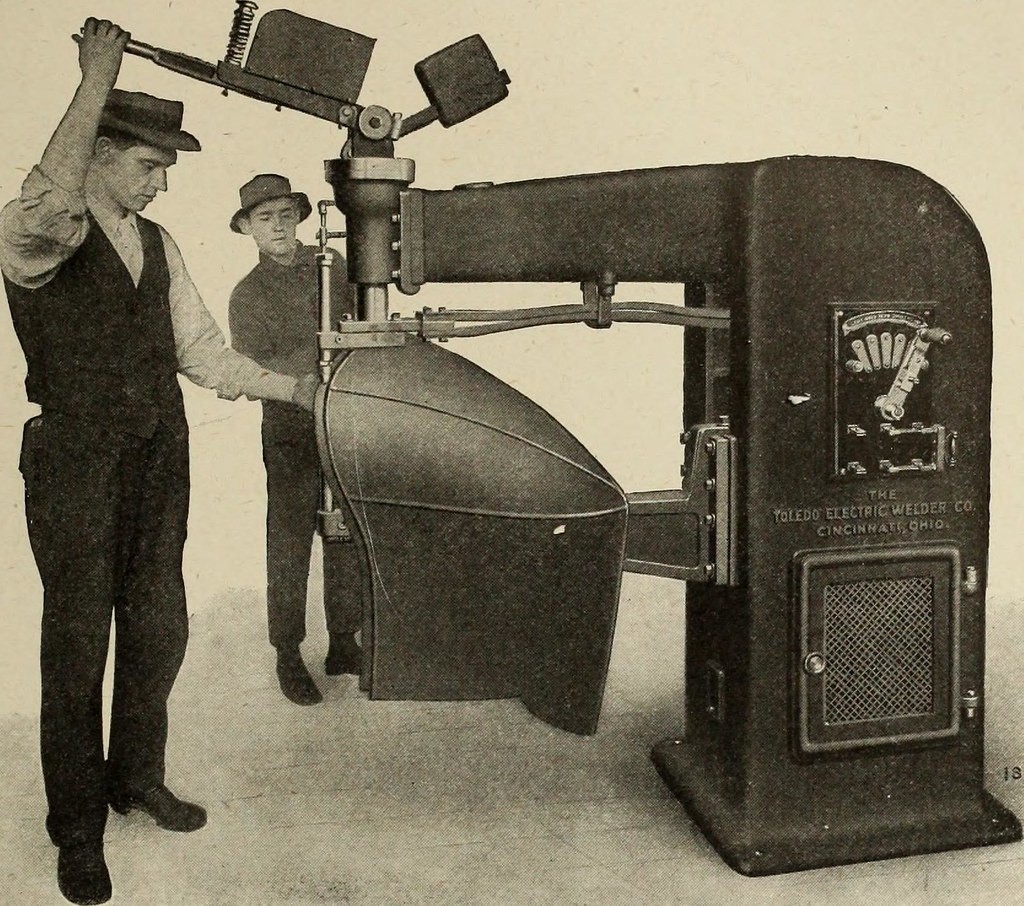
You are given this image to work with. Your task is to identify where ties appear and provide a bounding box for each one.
[118,220,141,289]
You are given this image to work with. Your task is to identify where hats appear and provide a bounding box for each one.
[229,174,312,235]
[100,89,201,151]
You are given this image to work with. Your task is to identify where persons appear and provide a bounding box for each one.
[0,17,321,906]
[216,173,360,704]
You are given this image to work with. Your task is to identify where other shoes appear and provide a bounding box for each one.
[109,783,207,832]
[277,646,321,706]
[57,834,112,906]
[325,632,362,676]
